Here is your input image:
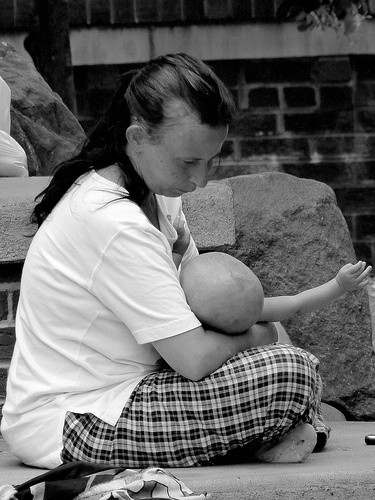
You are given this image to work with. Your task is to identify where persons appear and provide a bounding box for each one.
[1,50,331,466]
[180,251,371,333]
